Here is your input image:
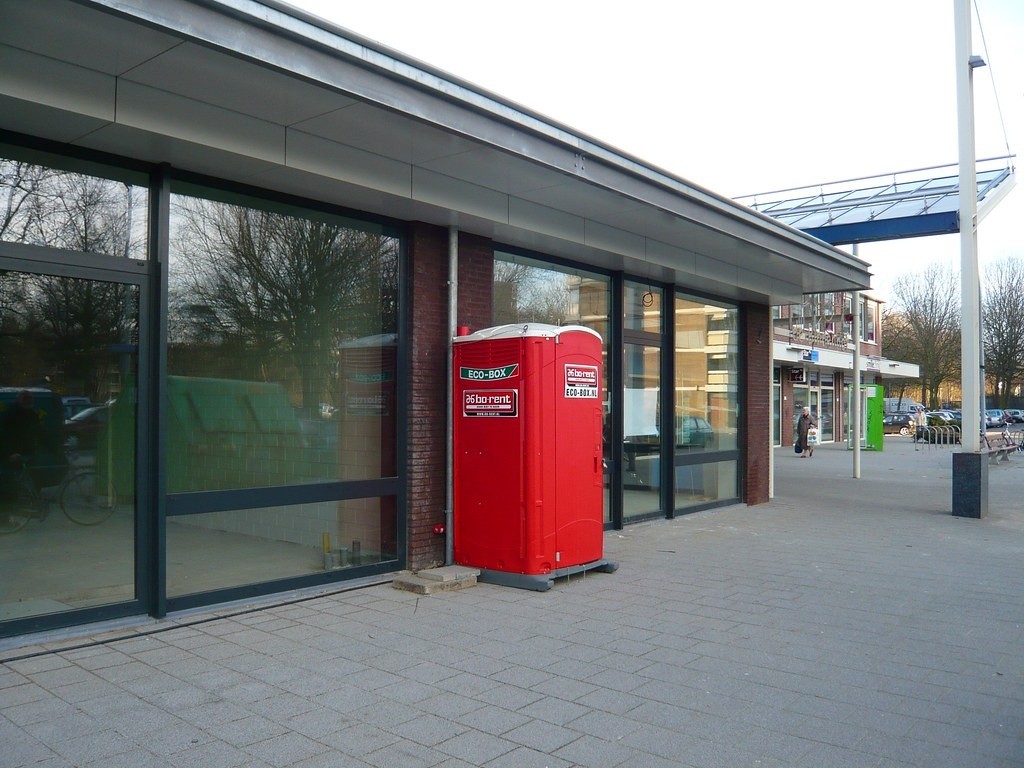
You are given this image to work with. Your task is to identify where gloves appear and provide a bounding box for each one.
[797,434,799,436]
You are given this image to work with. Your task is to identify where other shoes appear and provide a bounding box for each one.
[810,449,813,457]
[801,456,806,458]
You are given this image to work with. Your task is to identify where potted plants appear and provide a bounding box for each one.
[914,417,962,444]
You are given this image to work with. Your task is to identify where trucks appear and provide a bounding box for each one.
[883,398,925,415]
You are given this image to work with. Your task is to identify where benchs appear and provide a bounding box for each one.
[981,435,1019,465]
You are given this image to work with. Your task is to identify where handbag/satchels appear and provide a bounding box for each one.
[794,435,802,453]
[806,425,820,446]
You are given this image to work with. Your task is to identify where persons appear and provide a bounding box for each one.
[625,435,637,472]
[796,406,817,458]
[915,407,925,439]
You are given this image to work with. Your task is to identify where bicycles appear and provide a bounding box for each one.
[988,420,1024,452]
[0,447,119,535]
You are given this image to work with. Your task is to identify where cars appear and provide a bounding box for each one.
[59,404,107,453]
[925,409,961,427]
[980,409,1024,429]
[629,415,714,451]
[882,414,915,435]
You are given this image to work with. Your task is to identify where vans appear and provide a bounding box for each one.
[0,387,64,424]
[60,396,91,418]
